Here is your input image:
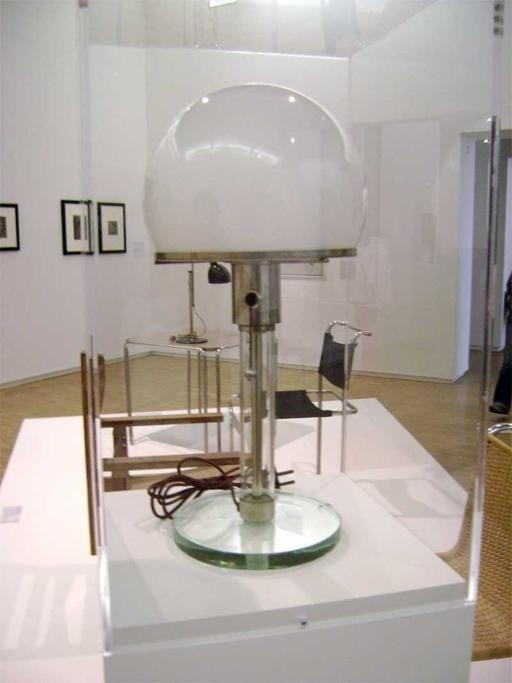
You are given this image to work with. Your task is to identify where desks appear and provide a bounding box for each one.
[121,326,242,454]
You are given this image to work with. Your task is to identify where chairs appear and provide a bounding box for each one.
[259,319,371,475]
[430,419,512,662]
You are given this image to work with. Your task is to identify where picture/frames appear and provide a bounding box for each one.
[60,197,125,255]
[0,202,19,251]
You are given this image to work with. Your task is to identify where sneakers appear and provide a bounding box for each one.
[489,403,509,415]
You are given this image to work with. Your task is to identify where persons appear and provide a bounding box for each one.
[486,268,512,414]
